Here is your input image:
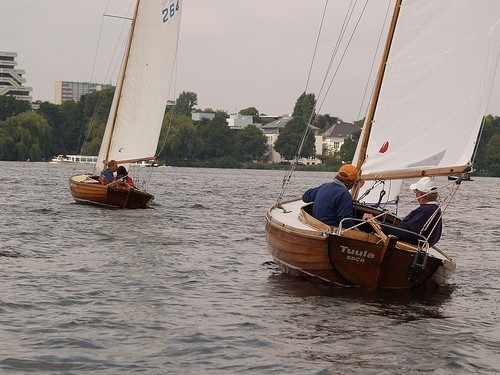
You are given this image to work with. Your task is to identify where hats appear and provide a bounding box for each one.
[117,166,126,175]
[338,164,359,180]
[410,177,439,193]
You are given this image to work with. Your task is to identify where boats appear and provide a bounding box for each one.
[51,155,158,168]
[448,175,473,182]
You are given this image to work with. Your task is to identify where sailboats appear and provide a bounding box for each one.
[265,0,500,293]
[69,0,183,209]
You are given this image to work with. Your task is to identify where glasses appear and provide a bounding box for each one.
[413,189,424,195]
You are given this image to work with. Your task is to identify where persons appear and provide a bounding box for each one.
[357,176,443,248]
[99,160,118,185]
[114,165,135,187]
[301,163,360,232]
